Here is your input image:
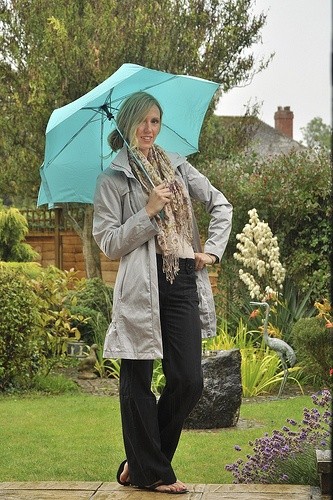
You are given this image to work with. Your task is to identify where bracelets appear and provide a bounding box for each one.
[206,253,215,265]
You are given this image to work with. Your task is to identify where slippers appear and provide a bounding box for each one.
[143,481,189,493]
[117,458,133,485]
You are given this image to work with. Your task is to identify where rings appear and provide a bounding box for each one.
[197,264,203,270]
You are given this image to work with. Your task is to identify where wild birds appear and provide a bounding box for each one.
[249,301,296,402]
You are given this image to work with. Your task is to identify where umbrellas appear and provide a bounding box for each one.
[35,63,221,210]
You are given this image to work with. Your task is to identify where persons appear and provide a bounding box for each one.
[90,90,234,495]
